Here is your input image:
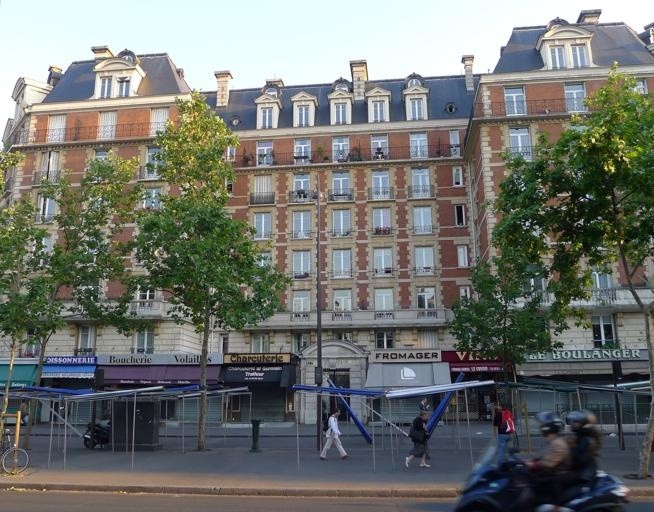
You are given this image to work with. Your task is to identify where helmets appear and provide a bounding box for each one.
[535,411,562,433]
[566,411,587,430]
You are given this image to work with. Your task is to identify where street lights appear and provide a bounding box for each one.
[313,174,325,450]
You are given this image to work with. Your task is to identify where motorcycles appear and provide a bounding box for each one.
[451,432,633,512]
[82,420,111,450]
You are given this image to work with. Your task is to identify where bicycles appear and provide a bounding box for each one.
[0,429,29,475]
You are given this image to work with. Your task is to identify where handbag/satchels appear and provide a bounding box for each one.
[504,417,516,434]
[411,429,425,443]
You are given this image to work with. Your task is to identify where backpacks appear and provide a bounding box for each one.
[324,414,334,432]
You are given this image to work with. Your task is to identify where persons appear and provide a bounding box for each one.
[405,412,433,468]
[319,408,351,461]
[521,410,570,511]
[418,397,429,409]
[19,400,29,426]
[550,409,600,511]
[490,399,516,466]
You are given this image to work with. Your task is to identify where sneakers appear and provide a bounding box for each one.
[405,456,410,468]
[420,463,431,468]
